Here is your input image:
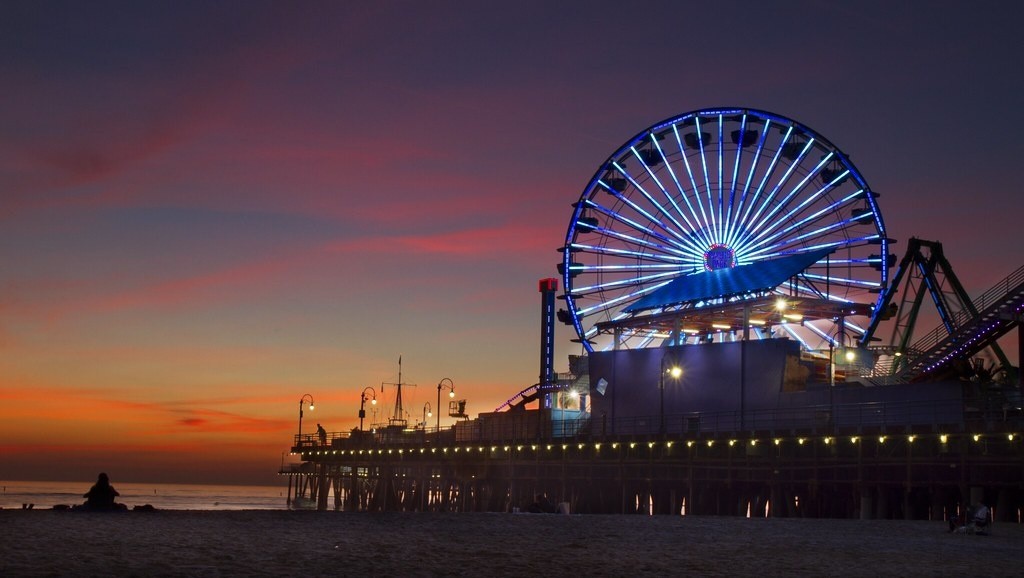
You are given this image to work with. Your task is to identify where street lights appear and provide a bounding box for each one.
[423,402,432,430]
[437,378,455,437]
[360,387,377,431]
[562,389,577,438]
[297,394,315,447]
[829,331,857,434]
[660,350,682,440]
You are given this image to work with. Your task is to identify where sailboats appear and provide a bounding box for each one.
[370,355,426,448]
